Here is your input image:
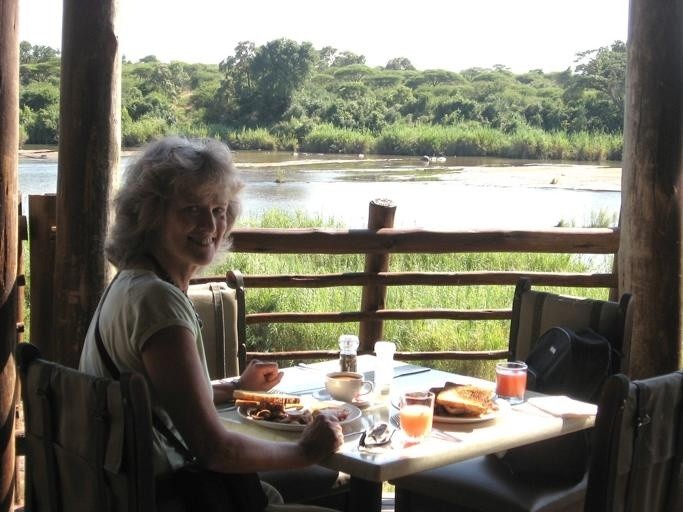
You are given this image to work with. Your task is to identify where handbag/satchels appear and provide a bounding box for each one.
[154,460,269,512]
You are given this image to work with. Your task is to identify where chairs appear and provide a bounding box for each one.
[187,269,247,380]
[14,342,156,512]
[388,278,633,511]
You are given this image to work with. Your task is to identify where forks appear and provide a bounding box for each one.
[390,413,463,443]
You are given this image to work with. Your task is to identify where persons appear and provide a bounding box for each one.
[76,133,344,512]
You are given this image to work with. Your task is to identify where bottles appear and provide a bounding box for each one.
[372,341,396,397]
[337,334,360,373]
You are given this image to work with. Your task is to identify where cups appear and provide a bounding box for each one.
[324,371,374,402]
[495,362,529,406]
[399,391,436,445]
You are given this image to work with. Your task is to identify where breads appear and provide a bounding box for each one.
[436,381,493,414]
[232,389,299,408]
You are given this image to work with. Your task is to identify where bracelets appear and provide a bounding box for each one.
[230,379,241,392]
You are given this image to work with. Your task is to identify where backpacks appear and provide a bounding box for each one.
[488,323,623,491]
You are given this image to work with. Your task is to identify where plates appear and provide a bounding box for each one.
[312,388,374,407]
[236,398,363,433]
[391,395,503,425]
